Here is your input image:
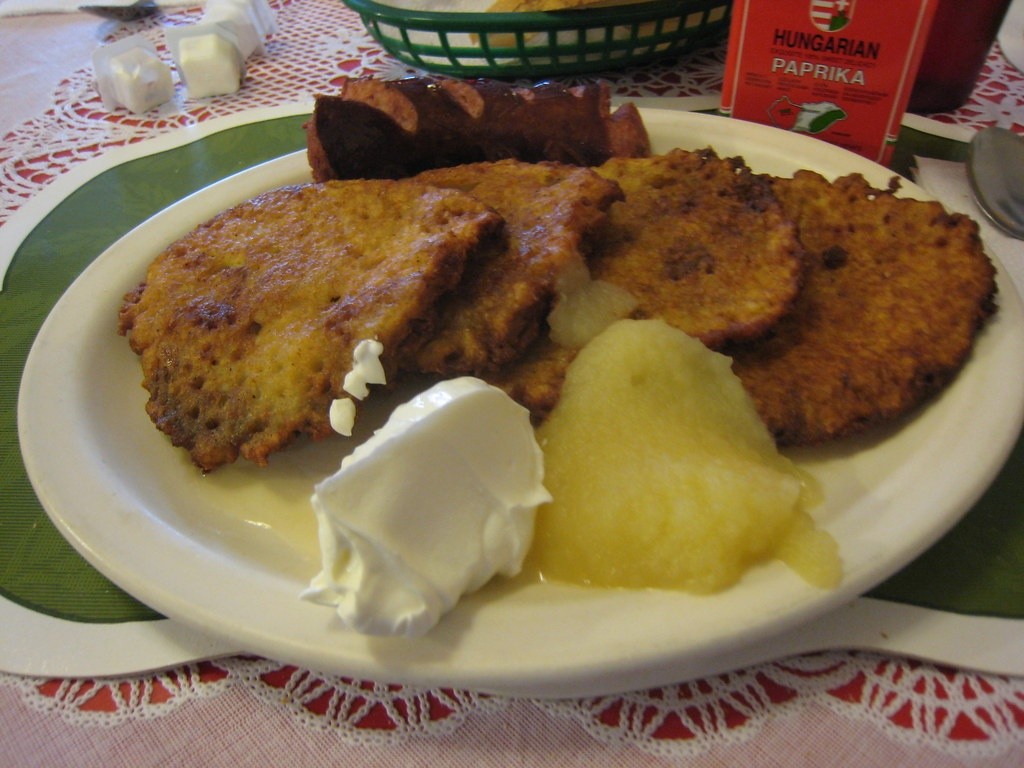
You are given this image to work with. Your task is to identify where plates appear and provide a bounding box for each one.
[14,103,1024,701]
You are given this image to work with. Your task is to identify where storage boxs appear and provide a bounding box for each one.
[719,1,938,167]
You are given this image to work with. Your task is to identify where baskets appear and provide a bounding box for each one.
[342,0,733,79]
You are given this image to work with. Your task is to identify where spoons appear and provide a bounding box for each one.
[77,0,159,20]
[964,126,1024,242]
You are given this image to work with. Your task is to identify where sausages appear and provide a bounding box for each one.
[304,75,650,182]
